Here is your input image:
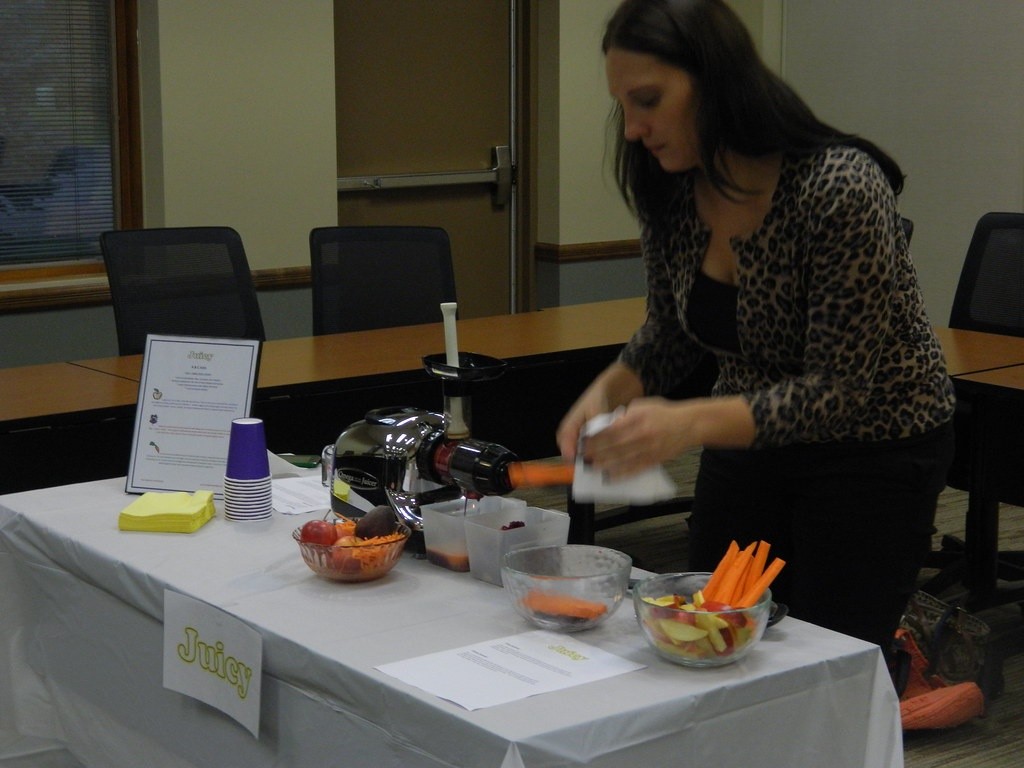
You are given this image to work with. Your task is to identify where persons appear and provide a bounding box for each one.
[554,0,956,701]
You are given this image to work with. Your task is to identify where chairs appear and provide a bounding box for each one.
[915,209,1024,610]
[99,224,321,458]
[309,225,526,451]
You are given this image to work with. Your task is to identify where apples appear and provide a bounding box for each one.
[300,520,367,573]
[641,594,753,662]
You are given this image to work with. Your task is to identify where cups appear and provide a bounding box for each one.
[224,418,273,523]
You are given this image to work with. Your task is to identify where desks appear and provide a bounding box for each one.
[1,294,1024,768]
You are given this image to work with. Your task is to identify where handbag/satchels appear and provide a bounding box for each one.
[899,589,990,684]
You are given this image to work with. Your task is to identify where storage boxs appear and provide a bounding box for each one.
[466,507,569,584]
[422,494,529,571]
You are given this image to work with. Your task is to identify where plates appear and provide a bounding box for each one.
[629,579,788,628]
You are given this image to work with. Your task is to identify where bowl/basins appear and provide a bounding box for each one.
[633,572,772,668]
[292,518,411,583]
[500,544,632,633]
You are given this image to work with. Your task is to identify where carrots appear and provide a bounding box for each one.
[507,463,574,487]
[521,594,610,619]
[701,538,786,609]
